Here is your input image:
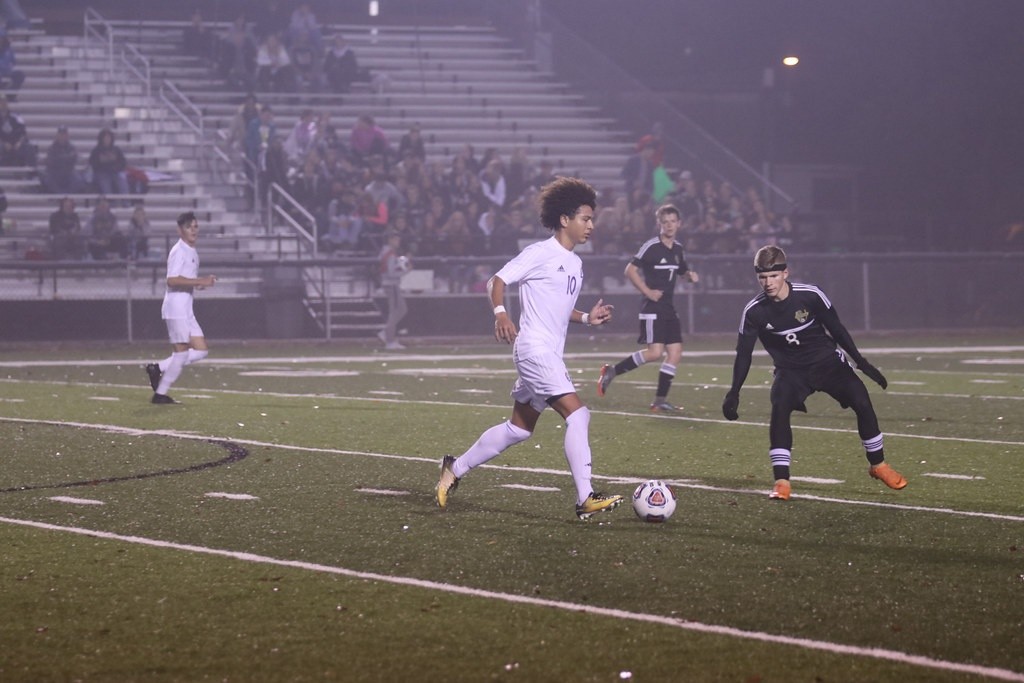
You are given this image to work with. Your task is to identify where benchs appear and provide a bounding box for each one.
[1,24,250,288]
[88,19,684,289]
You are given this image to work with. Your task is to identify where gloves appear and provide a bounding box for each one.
[857,358,887,389]
[722,392,739,420]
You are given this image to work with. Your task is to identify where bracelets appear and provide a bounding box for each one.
[582,312,590,326]
[494,305,505,315]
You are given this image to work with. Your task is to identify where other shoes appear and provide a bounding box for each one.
[386,338,404,350]
[377,330,387,343]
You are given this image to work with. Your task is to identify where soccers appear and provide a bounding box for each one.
[631,478,677,522]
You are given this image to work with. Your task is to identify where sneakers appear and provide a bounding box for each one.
[152,393,179,404]
[598,364,615,397]
[146,363,162,391]
[575,491,624,519]
[434,455,459,508]
[869,464,908,490]
[769,481,791,500]
[651,401,682,413]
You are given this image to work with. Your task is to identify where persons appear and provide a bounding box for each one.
[1,23,25,100]
[41,122,82,196]
[90,128,132,207]
[721,246,907,496]
[189,1,790,295]
[599,204,698,416]
[146,212,218,408]
[436,173,624,519]
[376,231,411,350]
[1,100,34,167]
[49,196,150,260]
